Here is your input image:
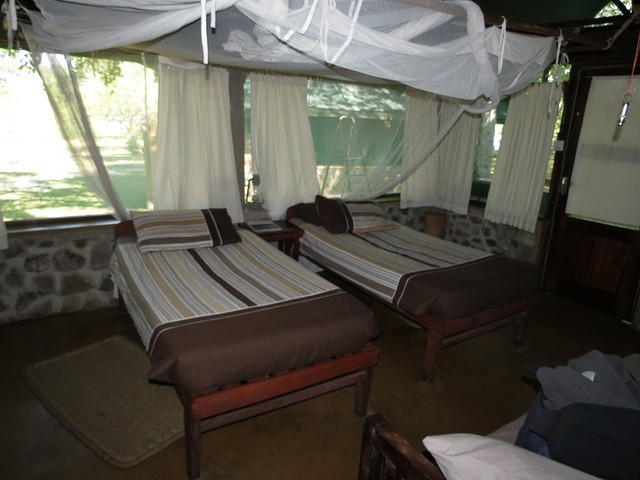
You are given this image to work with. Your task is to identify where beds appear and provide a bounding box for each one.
[109,218,382,480]
[358,412,528,480]
[286,202,539,382]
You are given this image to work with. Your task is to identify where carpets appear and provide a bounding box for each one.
[18,333,187,468]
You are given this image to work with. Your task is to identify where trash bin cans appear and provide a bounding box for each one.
[423,211,447,240]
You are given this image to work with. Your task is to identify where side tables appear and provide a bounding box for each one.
[236,219,305,262]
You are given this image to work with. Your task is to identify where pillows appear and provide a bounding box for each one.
[129,207,243,253]
[422,432,603,480]
[314,194,399,235]
